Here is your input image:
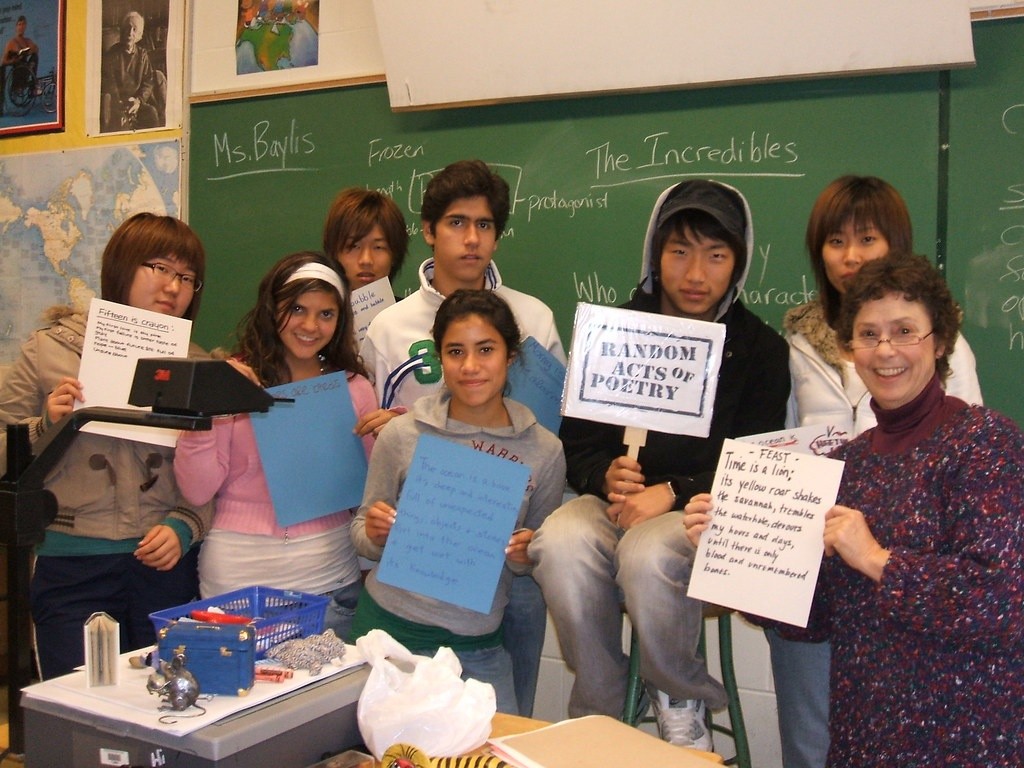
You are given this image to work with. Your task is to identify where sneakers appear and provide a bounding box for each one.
[644,679,714,753]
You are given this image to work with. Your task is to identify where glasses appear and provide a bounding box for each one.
[141,262,202,292]
[848,328,935,347]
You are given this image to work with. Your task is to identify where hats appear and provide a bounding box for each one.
[657,180,747,236]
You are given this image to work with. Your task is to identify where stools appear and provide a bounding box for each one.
[619,595,751,768]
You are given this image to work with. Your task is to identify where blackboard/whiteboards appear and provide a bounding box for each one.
[182,1,1024,490]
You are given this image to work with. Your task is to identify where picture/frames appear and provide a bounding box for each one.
[0,0,73,137]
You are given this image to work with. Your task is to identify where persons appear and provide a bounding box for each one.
[2,15,46,98]
[360,156,566,719]
[763,175,984,766]
[684,250,1024,766]
[350,287,566,719]
[322,179,407,381]
[102,12,163,130]
[3,212,219,685]
[172,250,411,630]
[529,175,791,752]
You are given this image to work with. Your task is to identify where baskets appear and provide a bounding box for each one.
[148,585,332,662]
